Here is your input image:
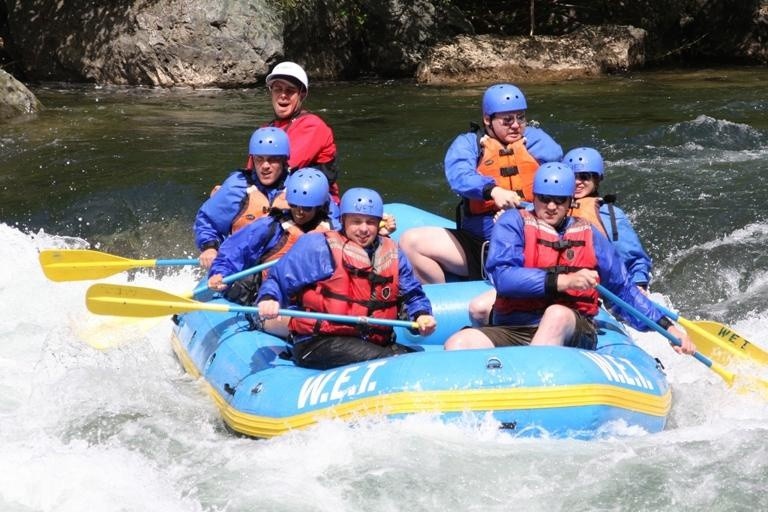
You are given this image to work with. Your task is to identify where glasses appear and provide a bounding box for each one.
[535,193,568,205]
[495,114,525,125]
[287,203,314,212]
[574,172,594,182]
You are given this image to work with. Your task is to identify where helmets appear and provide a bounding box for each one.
[285,167,330,208]
[531,161,576,197]
[248,126,290,160]
[560,146,605,182]
[265,61,309,120]
[481,84,527,144]
[339,187,384,220]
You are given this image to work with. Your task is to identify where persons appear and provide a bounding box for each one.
[446,161,696,354]
[254,187,437,371]
[210,62,339,207]
[398,85,563,284]
[207,168,397,305]
[193,127,329,269]
[467,147,650,329]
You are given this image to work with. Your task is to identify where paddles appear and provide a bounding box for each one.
[40,250,202,282]
[589,280,768,399]
[86,284,430,328]
[650,301,768,373]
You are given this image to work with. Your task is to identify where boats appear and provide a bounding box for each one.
[171,202,672,440]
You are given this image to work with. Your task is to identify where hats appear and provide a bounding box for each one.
[266,75,302,88]
